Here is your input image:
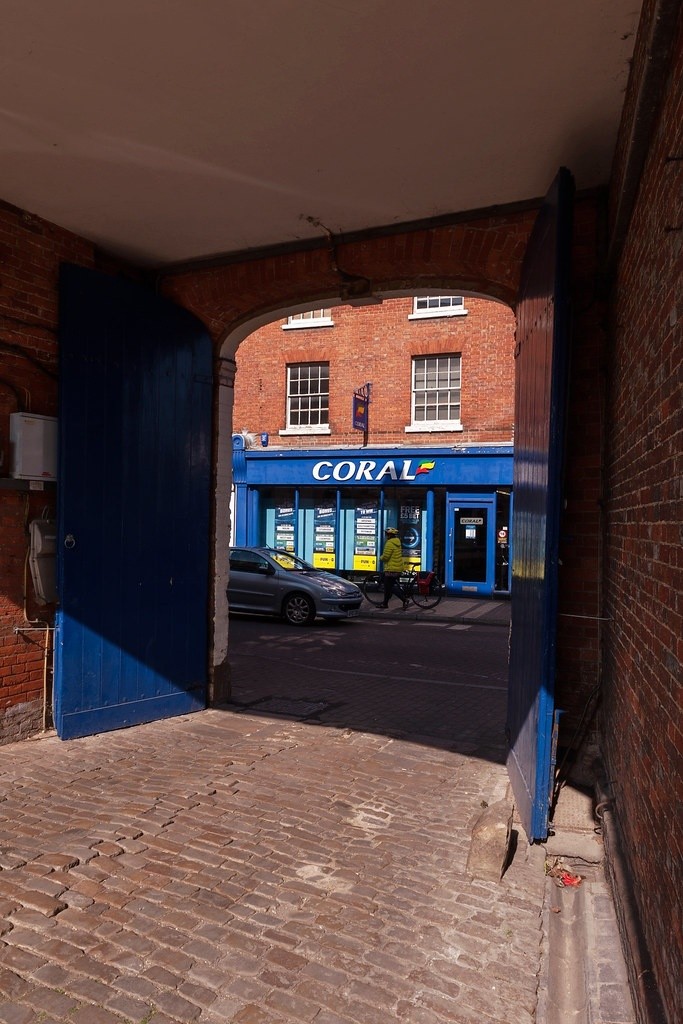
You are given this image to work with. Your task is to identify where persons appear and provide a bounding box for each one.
[375,526,409,611]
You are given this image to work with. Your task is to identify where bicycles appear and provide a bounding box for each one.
[361,562,443,609]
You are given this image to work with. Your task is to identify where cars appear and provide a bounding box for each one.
[228,546,365,625]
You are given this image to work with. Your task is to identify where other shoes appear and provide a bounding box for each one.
[376,602,388,609]
[402,601,410,611]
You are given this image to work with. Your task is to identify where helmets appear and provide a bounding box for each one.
[385,527,398,535]
